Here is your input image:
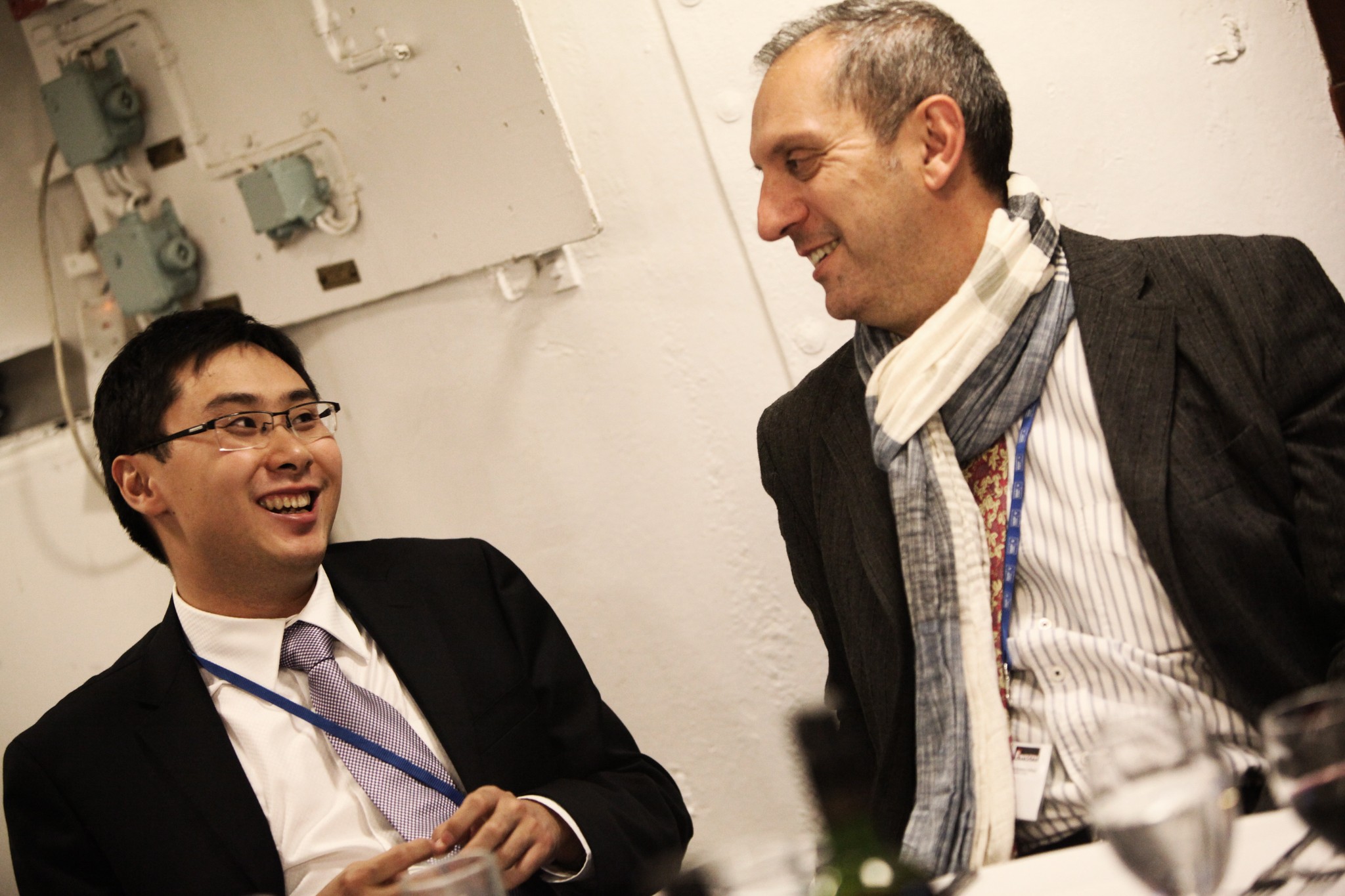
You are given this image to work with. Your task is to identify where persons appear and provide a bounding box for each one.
[748,4,1337,889]
[0,310,695,895]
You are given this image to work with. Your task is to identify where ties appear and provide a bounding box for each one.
[278,621,468,867]
[965,432,1008,735]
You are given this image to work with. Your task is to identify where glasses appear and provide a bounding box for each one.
[131,402,341,451]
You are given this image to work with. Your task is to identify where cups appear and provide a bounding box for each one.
[397,851,506,895]
[1084,701,1232,896]
[1261,679,1345,857]
